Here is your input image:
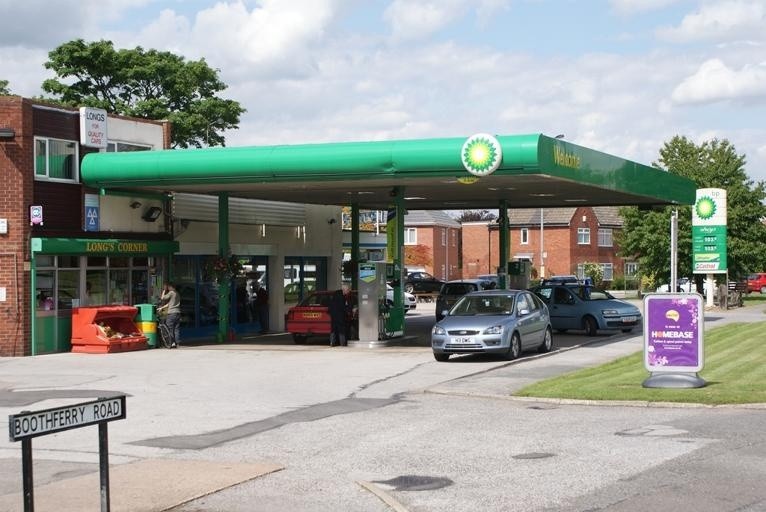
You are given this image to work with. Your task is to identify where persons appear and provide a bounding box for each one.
[254,282,270,332]
[326,283,352,346]
[155,279,184,348]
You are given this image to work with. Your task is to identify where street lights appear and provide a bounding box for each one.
[652,160,679,292]
[539,131,567,283]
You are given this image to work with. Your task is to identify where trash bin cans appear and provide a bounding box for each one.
[133,303,160,349]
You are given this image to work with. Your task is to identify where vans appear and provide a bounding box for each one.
[435,279,496,322]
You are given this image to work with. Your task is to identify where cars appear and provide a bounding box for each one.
[431,289,554,362]
[654,272,720,299]
[405,272,448,295]
[385,283,417,313]
[540,275,583,286]
[286,290,357,345]
[526,283,642,339]
[476,274,500,290]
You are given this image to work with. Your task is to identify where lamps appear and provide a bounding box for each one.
[142,207,161,223]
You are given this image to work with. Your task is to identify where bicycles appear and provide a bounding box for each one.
[154,303,172,349]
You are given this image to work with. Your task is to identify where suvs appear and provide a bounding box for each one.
[242,268,316,315]
[743,271,766,295]
